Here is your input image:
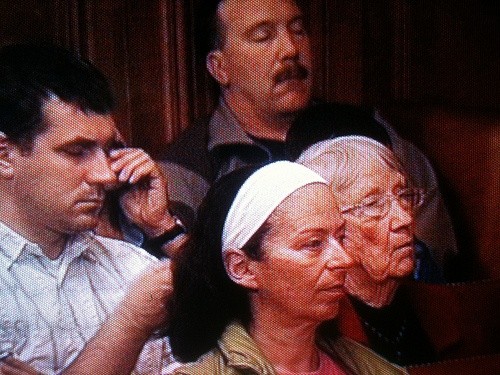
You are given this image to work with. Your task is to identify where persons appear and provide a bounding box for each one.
[1,0,500,375]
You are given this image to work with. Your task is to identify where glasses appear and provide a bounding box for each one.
[342,187,426,218]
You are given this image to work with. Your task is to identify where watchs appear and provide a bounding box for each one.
[144,215,187,249]
